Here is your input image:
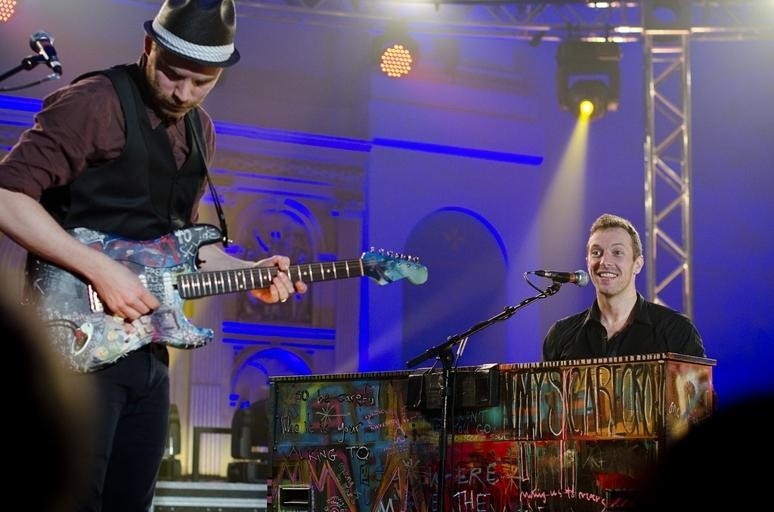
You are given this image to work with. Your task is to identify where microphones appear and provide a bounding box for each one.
[24,27,63,77]
[533,265,591,288]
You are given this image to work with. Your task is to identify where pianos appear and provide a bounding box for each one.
[264,353,718,511]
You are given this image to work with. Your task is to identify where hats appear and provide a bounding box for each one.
[143,0,241,68]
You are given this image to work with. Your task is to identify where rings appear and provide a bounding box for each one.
[280,298,288,303]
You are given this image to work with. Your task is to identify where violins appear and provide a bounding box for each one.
[24,222,428,375]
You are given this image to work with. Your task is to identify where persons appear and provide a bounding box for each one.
[543,212,708,360]
[0,0,308,512]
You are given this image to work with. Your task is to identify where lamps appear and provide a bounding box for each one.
[557,40,620,121]
[0,1,18,26]
[615,95,617,96]
[372,21,420,82]
